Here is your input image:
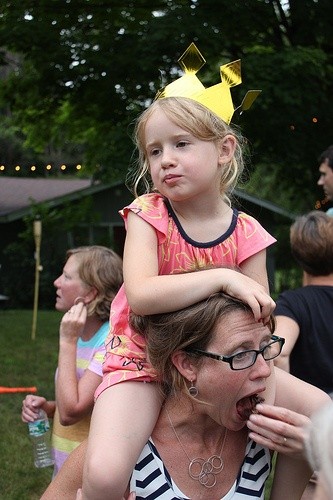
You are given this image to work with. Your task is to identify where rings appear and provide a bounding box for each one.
[281,437,288,447]
[68,310,74,313]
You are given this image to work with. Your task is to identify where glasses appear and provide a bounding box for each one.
[189,335,285,371]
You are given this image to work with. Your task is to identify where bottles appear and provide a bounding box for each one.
[28,408,54,467]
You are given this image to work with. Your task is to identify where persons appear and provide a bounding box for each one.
[38,263,332,500]
[271,211,333,391]
[314,143,333,213]
[18,243,126,480]
[78,94,333,499]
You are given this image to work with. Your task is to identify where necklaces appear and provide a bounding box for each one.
[163,402,228,490]
